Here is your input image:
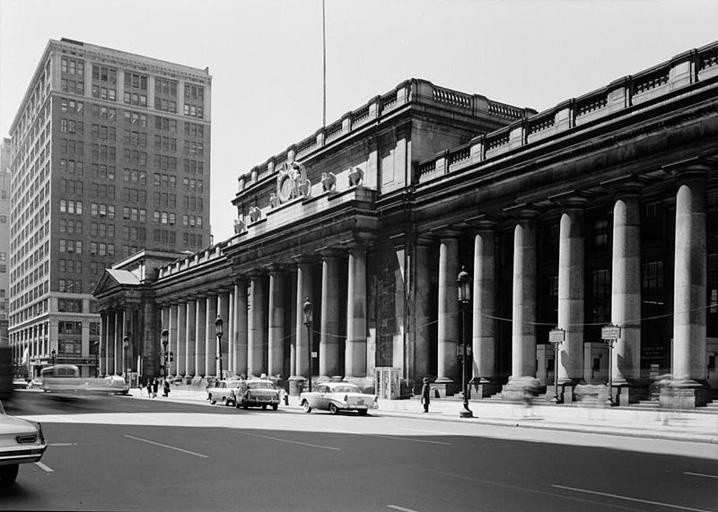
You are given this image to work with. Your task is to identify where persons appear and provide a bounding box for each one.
[162,379,169,396]
[421,376,430,413]
[146,378,152,398]
[151,377,159,398]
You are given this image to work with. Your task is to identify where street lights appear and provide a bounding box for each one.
[214,314,224,378]
[93,340,99,376]
[302,296,313,393]
[123,335,129,383]
[162,327,169,397]
[454,265,474,420]
[51,347,56,365]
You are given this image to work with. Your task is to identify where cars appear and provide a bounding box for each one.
[13,365,128,398]
[207,377,280,411]
[0,399,46,484]
[299,382,378,415]
